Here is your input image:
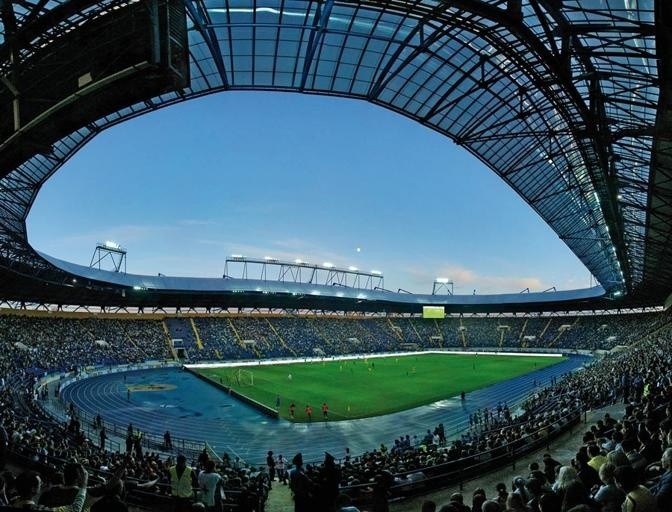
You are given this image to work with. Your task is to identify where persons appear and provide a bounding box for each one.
[0,304,670,510]
